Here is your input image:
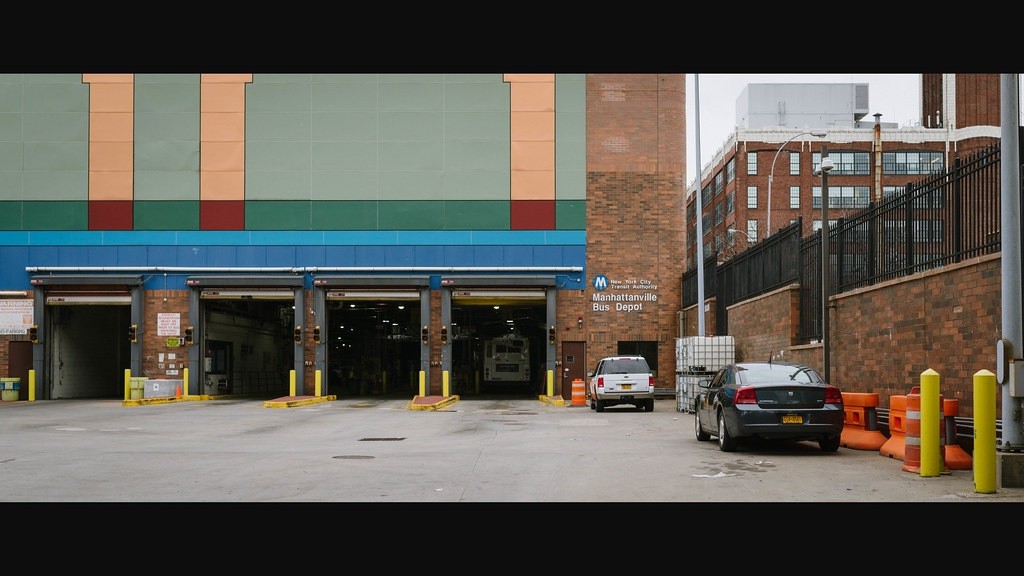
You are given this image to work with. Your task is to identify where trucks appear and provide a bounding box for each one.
[481,336,530,385]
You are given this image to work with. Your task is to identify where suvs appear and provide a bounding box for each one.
[587,355,655,412]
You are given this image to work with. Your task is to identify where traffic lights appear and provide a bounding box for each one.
[440,325,449,345]
[314,326,321,343]
[184,326,193,343]
[294,325,303,344]
[550,325,555,345]
[30,326,39,344]
[128,324,138,343]
[422,326,428,345]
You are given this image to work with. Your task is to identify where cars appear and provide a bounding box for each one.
[694,360,845,452]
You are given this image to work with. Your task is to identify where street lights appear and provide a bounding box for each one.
[765,131,827,237]
